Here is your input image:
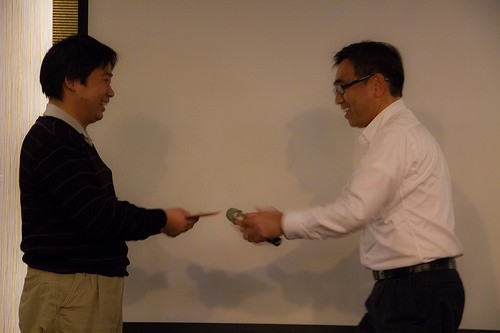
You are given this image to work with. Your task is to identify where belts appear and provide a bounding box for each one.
[372,259,456,281]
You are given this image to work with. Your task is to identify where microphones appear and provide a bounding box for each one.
[224,206,283,249]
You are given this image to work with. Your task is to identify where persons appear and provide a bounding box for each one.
[18,32,200,332]
[238,39,466,333]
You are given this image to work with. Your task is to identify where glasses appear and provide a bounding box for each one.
[333,74,390,94]
